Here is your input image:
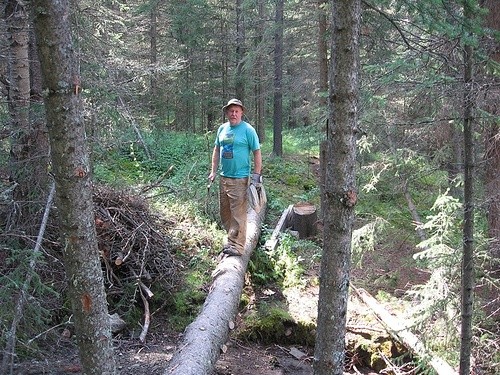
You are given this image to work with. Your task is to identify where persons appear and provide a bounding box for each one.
[209,99,262,258]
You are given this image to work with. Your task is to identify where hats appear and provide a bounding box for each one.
[222,98,248,114]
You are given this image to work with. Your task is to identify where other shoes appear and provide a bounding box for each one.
[221,244,243,256]
